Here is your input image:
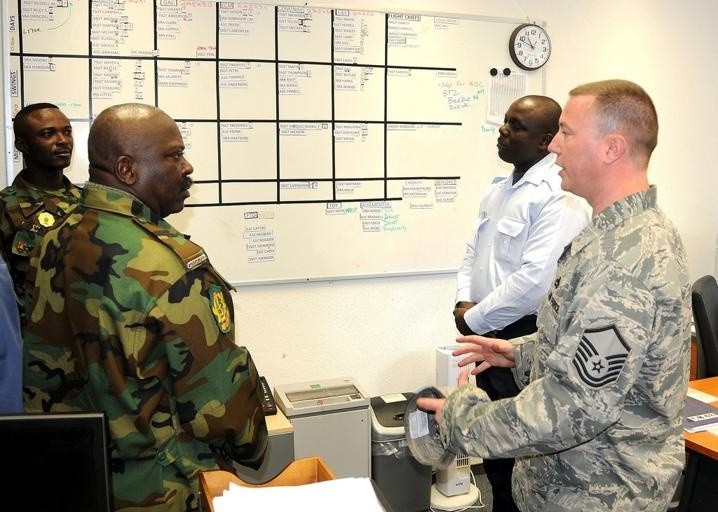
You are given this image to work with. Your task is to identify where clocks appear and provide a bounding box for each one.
[509,24,552,70]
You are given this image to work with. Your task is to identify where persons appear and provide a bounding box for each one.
[452,94,593,511]
[416,84,687,511]
[20,103,268,512]
[0,102,88,328]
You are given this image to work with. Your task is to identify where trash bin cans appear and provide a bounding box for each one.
[370,393,432,512]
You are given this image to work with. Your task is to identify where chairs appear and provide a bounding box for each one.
[691,276,718,377]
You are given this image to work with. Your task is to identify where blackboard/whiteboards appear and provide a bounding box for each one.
[3,0,541,287]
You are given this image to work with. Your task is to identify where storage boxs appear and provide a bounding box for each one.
[198,456,334,512]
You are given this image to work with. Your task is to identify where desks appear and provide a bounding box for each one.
[681,376,718,512]
